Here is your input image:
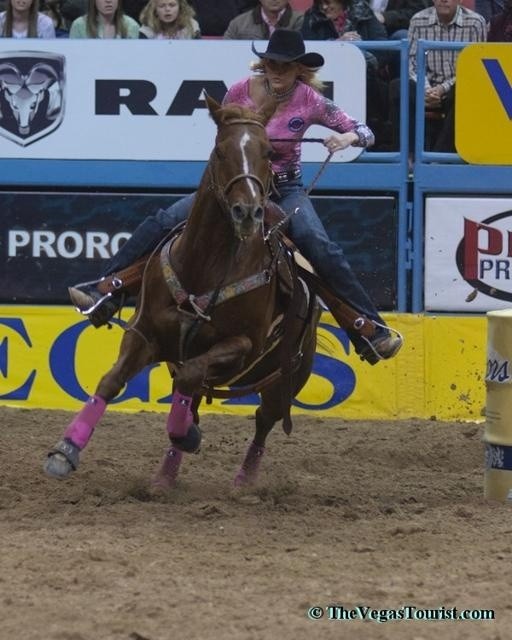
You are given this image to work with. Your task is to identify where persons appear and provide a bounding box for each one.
[67,27,403,367]
[1,1,511,164]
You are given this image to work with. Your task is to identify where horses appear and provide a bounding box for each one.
[43,87,321,506]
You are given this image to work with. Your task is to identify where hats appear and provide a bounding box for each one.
[251,28,324,67]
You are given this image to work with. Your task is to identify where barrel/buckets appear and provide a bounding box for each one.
[483,308,512,505]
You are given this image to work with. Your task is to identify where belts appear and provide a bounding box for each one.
[276,169,303,184]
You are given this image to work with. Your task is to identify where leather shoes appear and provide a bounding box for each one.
[68,286,113,328]
[360,336,402,365]
